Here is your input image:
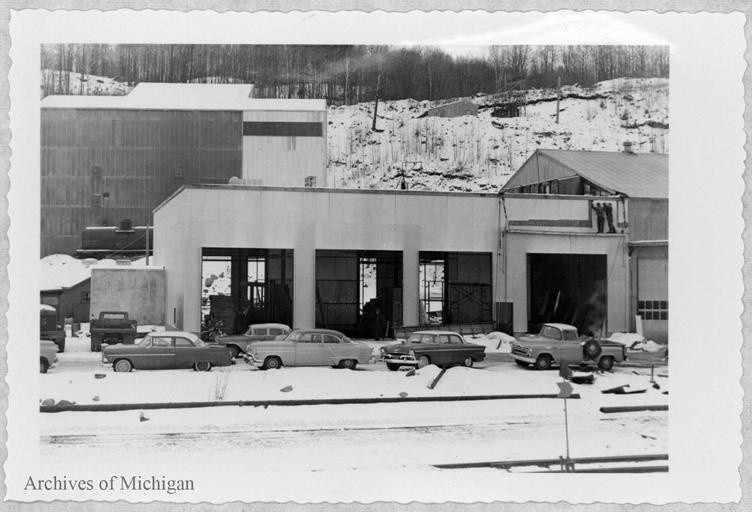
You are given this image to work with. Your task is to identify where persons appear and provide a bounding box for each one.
[603,202,616,233]
[591,202,605,233]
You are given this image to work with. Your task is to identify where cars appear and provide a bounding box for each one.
[41,340,59,373]
[101,331,230,374]
[379,331,485,370]
[218,324,371,369]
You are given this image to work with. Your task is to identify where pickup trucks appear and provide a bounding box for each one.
[509,322,627,370]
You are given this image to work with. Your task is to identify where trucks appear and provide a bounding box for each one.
[89,311,137,352]
[40,310,66,352]
